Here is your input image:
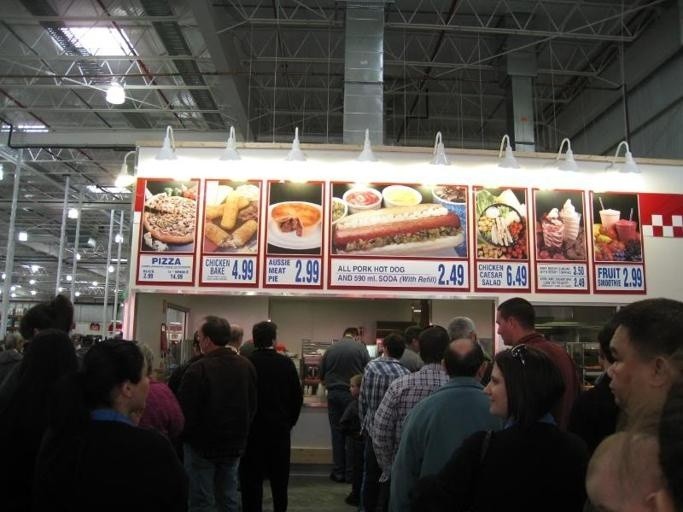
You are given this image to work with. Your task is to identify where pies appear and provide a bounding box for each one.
[270,202,322,237]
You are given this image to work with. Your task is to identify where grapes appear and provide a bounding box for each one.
[612,238,641,261]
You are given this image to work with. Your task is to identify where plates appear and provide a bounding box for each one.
[478,203,524,247]
[267,199,321,250]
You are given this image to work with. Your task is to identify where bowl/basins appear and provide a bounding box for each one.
[431,186,468,224]
[331,185,422,230]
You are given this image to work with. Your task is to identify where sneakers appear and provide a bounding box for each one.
[345,496,358,506]
[329,472,345,482]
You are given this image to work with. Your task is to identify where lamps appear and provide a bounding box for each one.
[550,137,580,173]
[153,124,178,161]
[427,128,450,167]
[603,139,643,175]
[285,127,306,163]
[112,149,138,189]
[494,133,523,171]
[352,127,376,162]
[218,125,241,162]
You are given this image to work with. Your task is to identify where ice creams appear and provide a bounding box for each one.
[559,199,580,241]
[540,207,563,249]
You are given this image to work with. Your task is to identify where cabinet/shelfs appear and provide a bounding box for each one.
[534,320,609,390]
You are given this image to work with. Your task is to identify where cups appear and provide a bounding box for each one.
[616,221,638,242]
[559,213,582,244]
[599,210,620,227]
[541,215,563,251]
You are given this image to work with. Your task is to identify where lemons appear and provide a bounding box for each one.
[597,234,612,243]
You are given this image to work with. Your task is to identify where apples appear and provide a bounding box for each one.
[599,226,617,240]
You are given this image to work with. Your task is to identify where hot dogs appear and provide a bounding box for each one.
[332,203,464,257]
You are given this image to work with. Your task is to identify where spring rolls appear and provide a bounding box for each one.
[204,193,257,249]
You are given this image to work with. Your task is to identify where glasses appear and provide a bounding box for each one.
[511,344,527,366]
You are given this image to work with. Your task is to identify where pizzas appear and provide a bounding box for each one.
[144,192,197,245]
